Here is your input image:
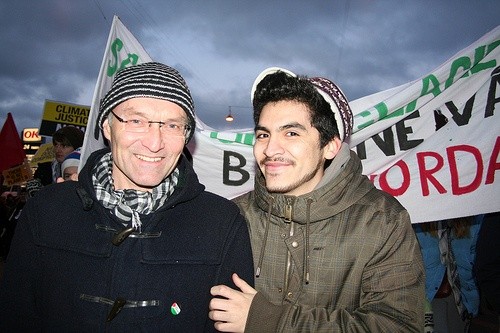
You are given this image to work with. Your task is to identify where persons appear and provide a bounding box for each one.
[412,212,500,333]
[0,126,86,243]
[209,66,426,333]
[0,61,255,333]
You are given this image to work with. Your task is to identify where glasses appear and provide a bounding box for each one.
[109,109,192,140]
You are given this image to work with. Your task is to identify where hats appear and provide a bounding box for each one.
[97,61,196,145]
[252,67,354,144]
[61,145,82,178]
